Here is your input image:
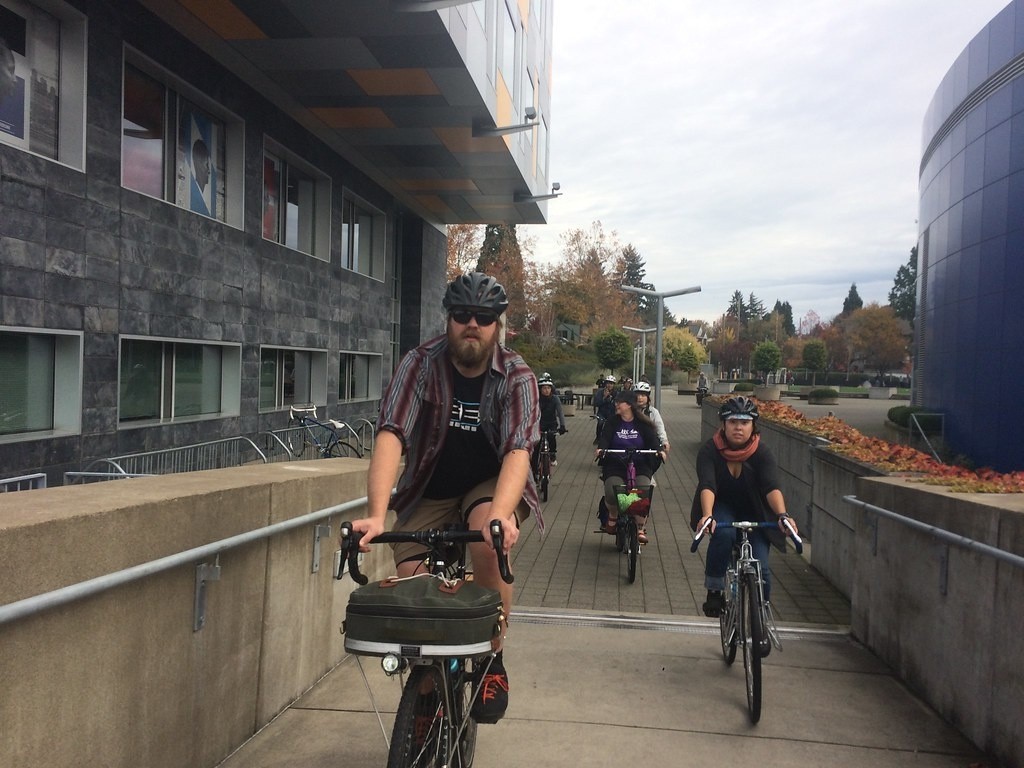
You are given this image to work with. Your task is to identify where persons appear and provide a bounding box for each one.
[590,370,670,543]
[339,272,541,768]
[532,377,566,487]
[542,372,559,395]
[690,397,797,657]
[697,372,708,389]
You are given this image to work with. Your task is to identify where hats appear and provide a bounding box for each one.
[724,412,754,421]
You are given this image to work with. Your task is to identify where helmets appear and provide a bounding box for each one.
[604,376,617,384]
[632,382,651,393]
[615,391,637,404]
[442,270,509,314]
[718,397,759,422]
[623,378,632,387]
[537,377,554,385]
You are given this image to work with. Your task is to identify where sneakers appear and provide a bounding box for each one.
[467,663,510,725]
[409,705,446,767]
[707,588,725,616]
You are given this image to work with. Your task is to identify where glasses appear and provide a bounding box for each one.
[448,307,498,326]
[615,401,625,406]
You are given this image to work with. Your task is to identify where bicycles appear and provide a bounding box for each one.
[337,519,514,768]
[595,448,666,583]
[356,398,381,451]
[590,414,612,443]
[697,387,708,407]
[540,429,568,501]
[287,404,362,459]
[690,516,803,723]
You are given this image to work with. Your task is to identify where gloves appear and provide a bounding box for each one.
[559,425,566,435]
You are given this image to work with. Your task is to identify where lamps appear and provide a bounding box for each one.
[514,183,562,204]
[471,106,540,138]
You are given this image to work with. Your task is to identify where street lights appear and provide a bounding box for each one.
[622,326,666,382]
[620,285,701,415]
[633,339,647,386]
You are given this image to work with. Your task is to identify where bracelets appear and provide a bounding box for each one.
[776,511,790,518]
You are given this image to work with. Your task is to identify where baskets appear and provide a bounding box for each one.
[613,484,655,516]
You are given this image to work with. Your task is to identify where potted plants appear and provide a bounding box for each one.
[749,342,781,400]
[808,387,839,405]
[799,339,827,400]
[733,382,756,397]
[884,403,943,449]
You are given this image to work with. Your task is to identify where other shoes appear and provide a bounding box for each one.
[607,517,615,534]
[638,531,648,543]
[551,458,557,466]
